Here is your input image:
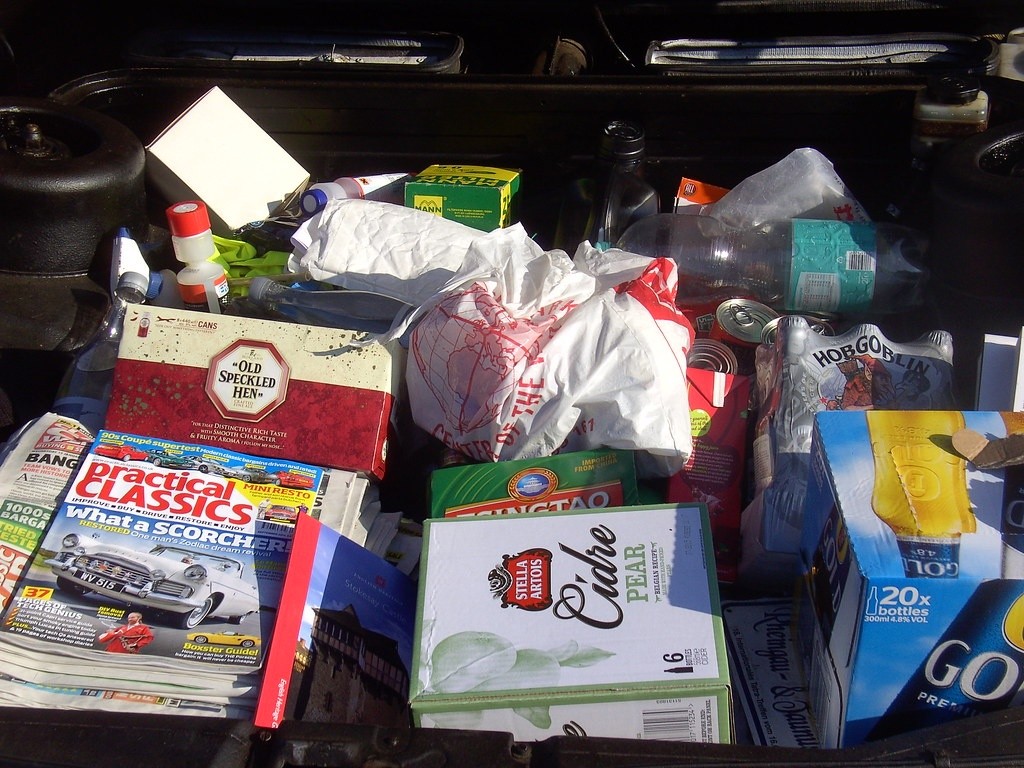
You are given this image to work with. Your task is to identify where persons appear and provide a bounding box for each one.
[98,612,154,655]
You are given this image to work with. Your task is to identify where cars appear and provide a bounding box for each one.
[50,540,260,631]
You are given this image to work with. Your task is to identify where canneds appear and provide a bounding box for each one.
[685,298,836,376]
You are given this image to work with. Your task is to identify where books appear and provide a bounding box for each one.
[0,428,327,708]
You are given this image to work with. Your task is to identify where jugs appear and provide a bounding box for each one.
[551,118,660,259]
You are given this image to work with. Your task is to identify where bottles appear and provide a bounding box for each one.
[302,174,417,212]
[52,271,149,432]
[745,317,956,559]
[601,216,920,315]
[166,202,229,314]
[247,278,427,349]
[864,410,977,578]
